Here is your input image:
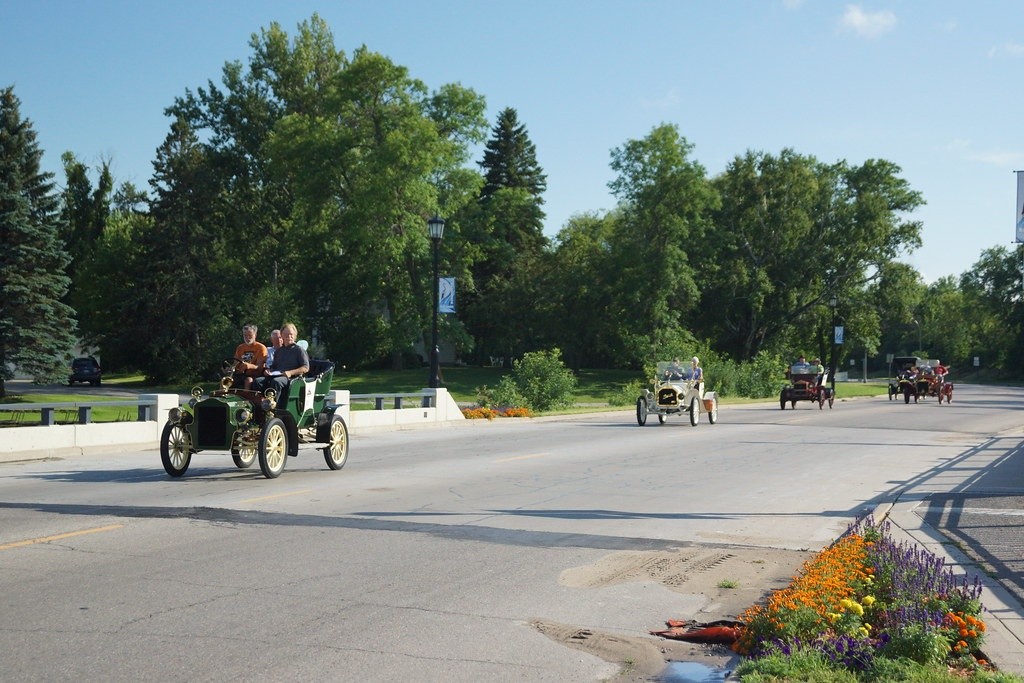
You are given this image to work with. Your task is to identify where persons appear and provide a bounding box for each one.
[792,355,811,372]
[229,321,310,407]
[663,357,685,379]
[903,358,948,382]
[684,356,702,382]
[807,358,824,375]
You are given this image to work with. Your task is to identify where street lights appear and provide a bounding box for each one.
[428,208,447,388]
[830,292,837,368]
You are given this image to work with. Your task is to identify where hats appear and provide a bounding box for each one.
[813,358,821,365]
[798,355,805,359]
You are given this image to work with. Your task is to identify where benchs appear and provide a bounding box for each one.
[276,360,335,429]
[0,401,75,426]
[323,394,334,406]
[395,392,434,408]
[350,394,395,409]
[75,399,155,424]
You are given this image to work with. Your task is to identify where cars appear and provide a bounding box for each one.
[780,364,835,410]
[636,361,719,427]
[68,357,102,387]
[160,356,349,479]
[888,360,955,405]
